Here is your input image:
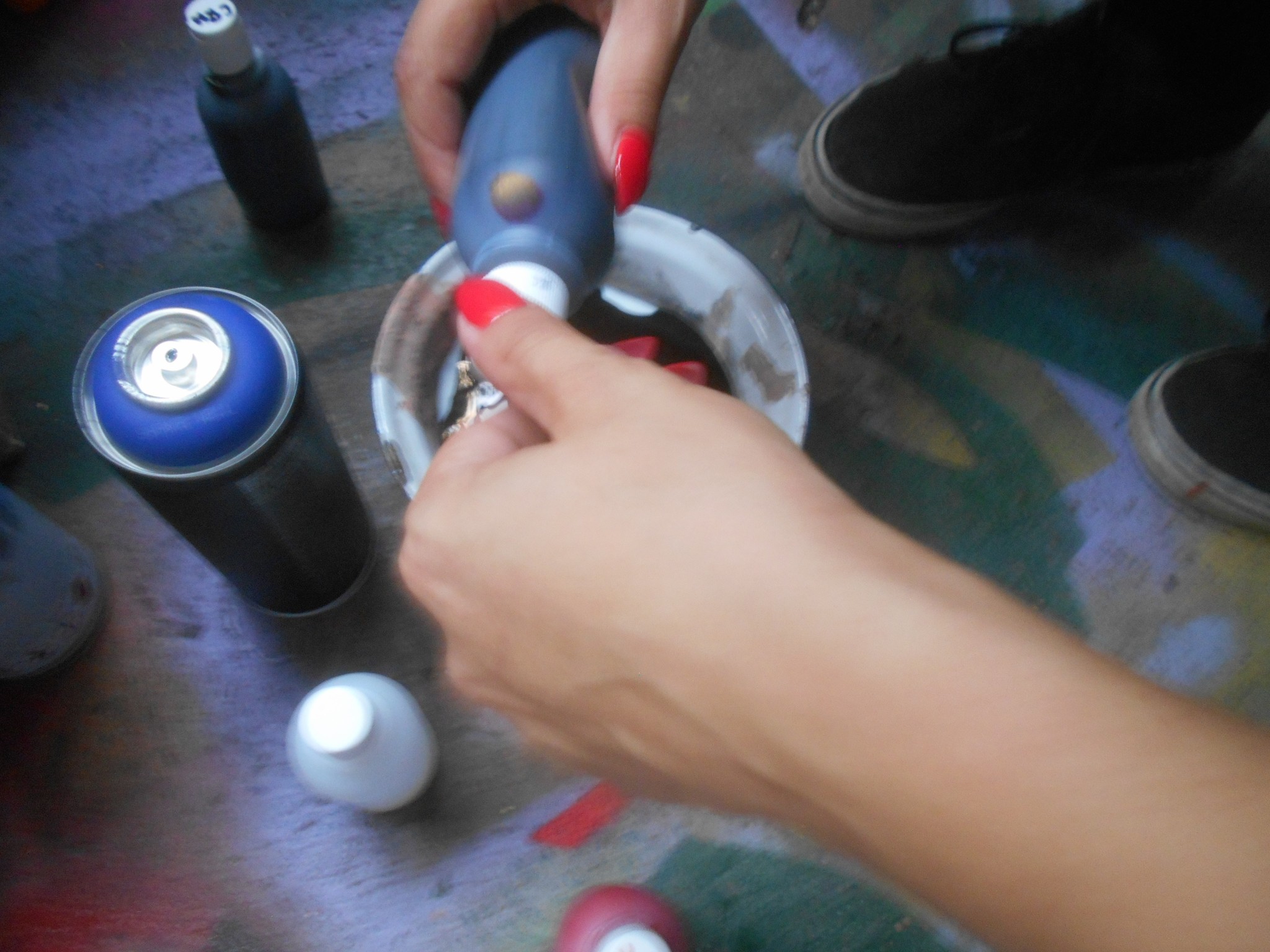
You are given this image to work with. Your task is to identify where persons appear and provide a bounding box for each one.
[395,0,1270,952]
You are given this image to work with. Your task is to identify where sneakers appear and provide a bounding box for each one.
[1125,343,1270,533]
[793,14,1228,245]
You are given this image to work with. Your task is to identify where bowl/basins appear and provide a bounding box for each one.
[370,204,811,501]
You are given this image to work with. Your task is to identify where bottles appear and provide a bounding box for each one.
[450,3,614,322]
[0,492,96,694]
[284,670,437,810]
[556,881,692,952]
[71,288,379,620]
[180,0,327,238]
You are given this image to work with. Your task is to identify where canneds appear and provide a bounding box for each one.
[0,485,104,682]
